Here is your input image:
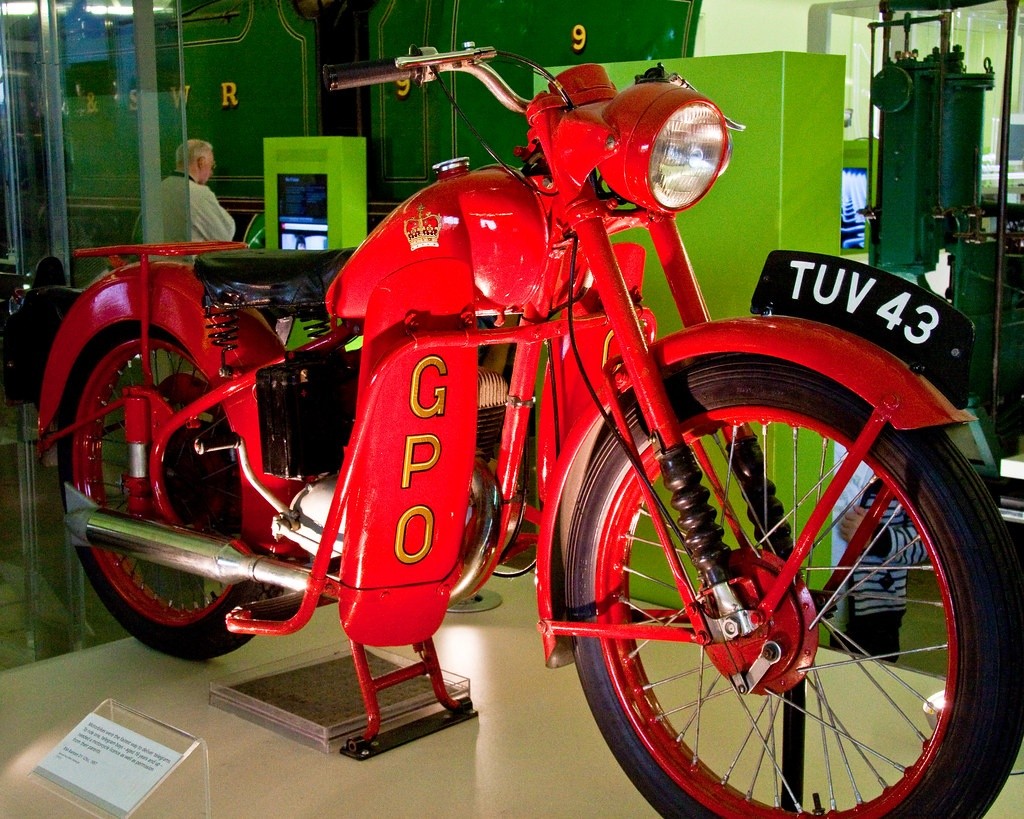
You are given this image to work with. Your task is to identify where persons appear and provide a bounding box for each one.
[837,471,931,664]
[133,137,234,265]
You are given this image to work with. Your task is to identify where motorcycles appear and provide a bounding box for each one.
[38,46,1023,819]
